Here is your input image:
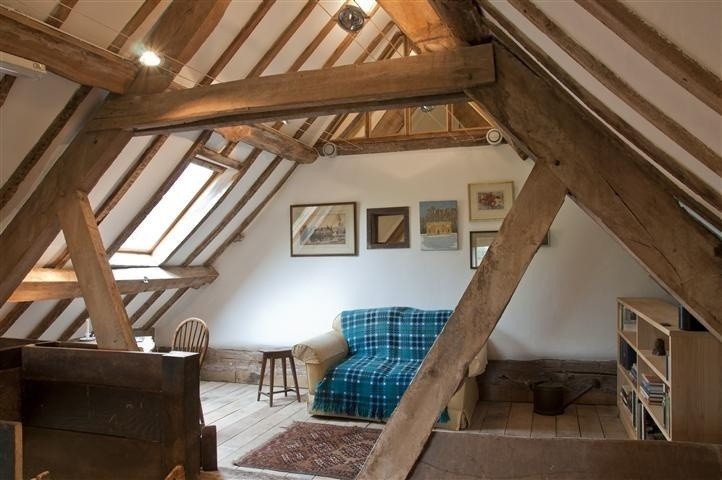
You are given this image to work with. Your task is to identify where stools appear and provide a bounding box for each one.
[255,347,302,407]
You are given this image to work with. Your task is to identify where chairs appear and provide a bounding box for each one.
[169,317,209,372]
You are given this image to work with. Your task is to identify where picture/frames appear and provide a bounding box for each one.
[466,177,553,271]
[417,197,460,253]
[288,201,358,257]
[364,204,412,250]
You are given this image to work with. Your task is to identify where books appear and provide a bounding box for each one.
[618,305,670,440]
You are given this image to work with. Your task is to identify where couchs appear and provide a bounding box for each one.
[291,304,491,433]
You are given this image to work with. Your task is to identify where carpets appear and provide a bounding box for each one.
[231,416,401,479]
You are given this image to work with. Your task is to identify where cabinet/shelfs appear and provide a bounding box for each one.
[612,294,722,448]
